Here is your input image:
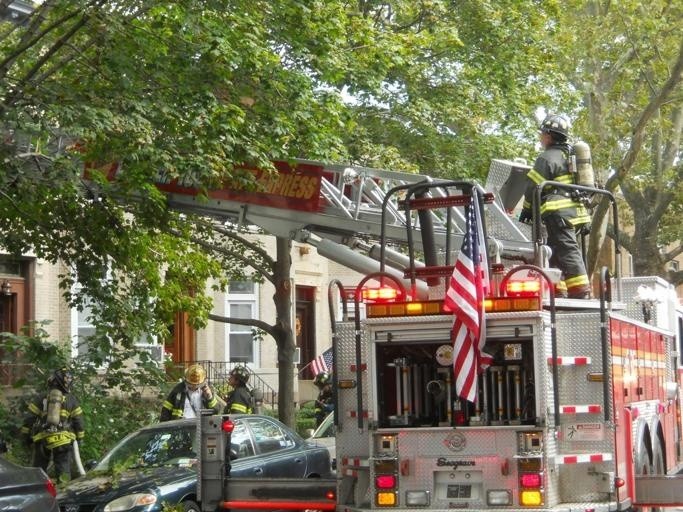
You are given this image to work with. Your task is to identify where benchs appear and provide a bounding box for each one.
[311,349,333,376]
[445,188,495,405]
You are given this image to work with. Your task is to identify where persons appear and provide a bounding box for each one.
[222,365,252,413]
[22,373,85,483]
[517,112,593,301]
[311,370,337,428]
[160,364,221,422]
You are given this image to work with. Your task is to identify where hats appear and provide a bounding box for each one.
[301,410,336,471]
[0,456,62,512]
[54,413,332,511]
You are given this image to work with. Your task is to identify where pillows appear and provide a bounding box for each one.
[185,365,207,385]
[233,366,250,384]
[541,115,568,137]
[54,366,73,392]
[313,373,331,385]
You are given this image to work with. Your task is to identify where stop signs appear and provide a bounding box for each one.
[2,122,682,510]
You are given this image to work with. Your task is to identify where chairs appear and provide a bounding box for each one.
[555,285,590,298]
